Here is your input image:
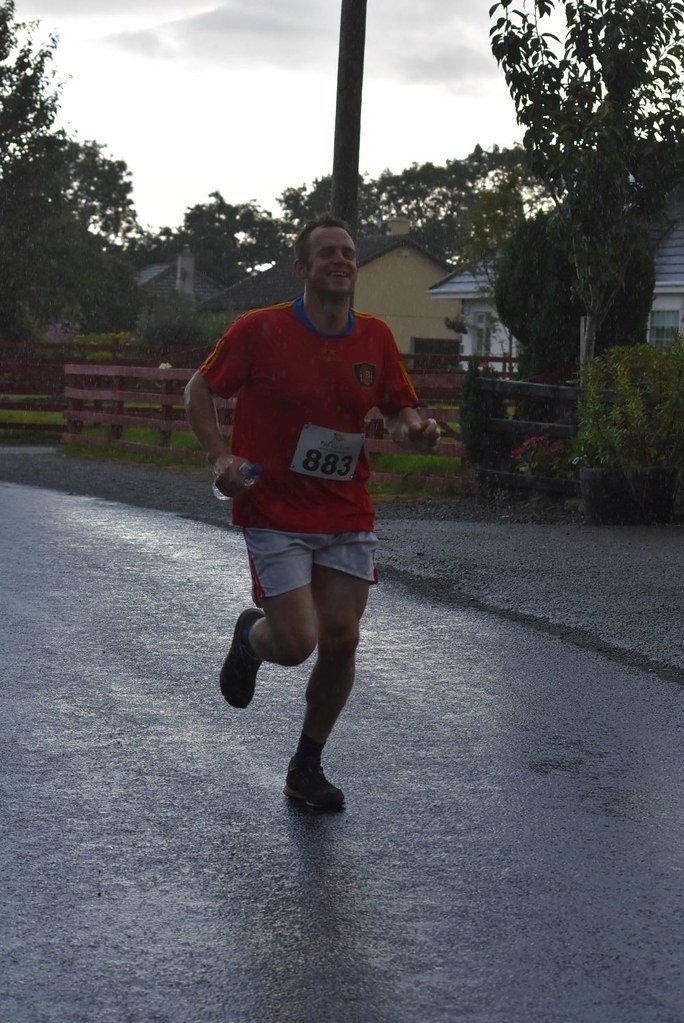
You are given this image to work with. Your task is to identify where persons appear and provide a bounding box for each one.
[183,217,442,814]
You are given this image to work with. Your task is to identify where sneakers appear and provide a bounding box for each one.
[219,607,264,709]
[282,757,345,807]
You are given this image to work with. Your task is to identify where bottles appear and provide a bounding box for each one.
[213,463,262,501]
